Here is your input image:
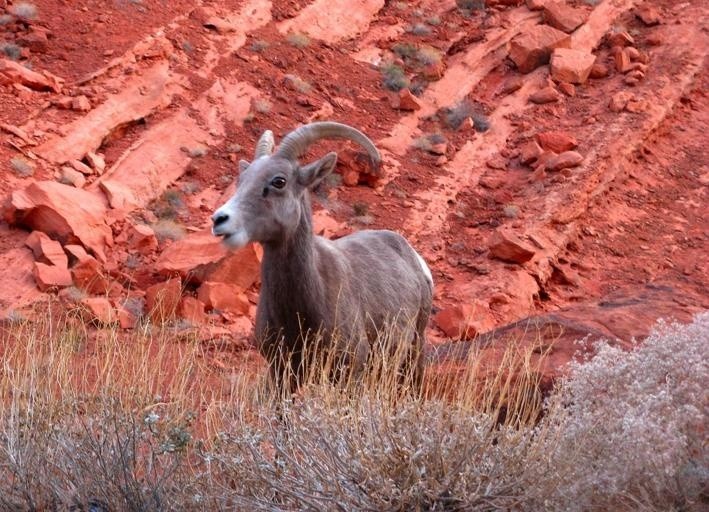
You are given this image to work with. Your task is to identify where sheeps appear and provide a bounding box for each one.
[210,122,435,424]
